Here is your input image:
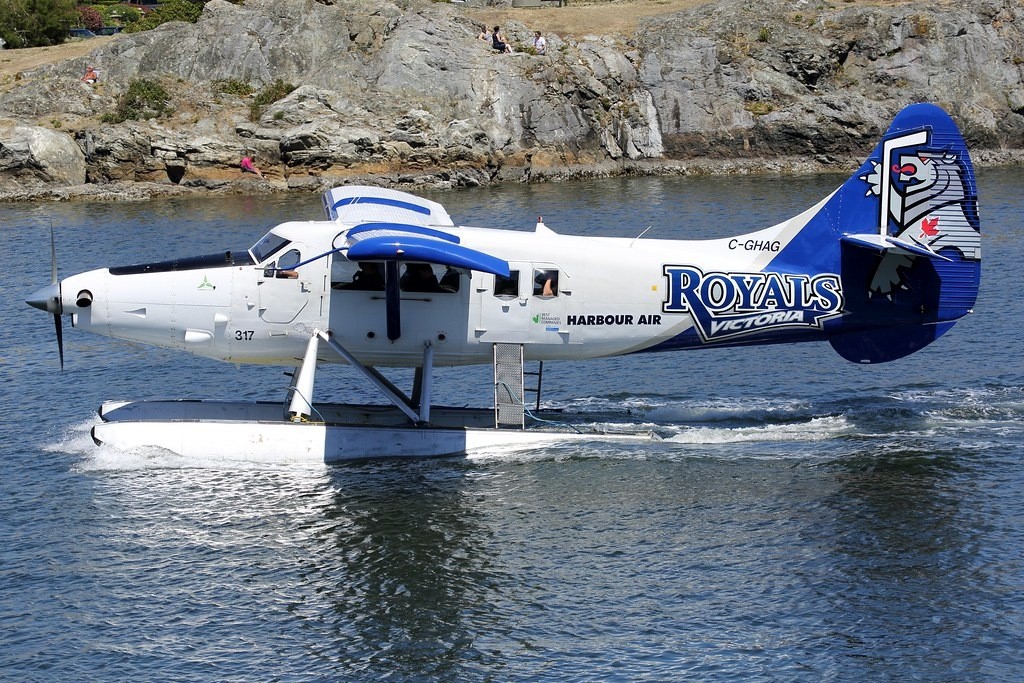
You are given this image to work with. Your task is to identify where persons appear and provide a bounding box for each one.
[400,262,460,292]
[542,271,558,296]
[478,24,512,54]
[346,260,382,291]
[81,66,96,84]
[241,152,266,179]
[529,31,546,55]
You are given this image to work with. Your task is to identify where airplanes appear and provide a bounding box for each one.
[24,100,985,467]
